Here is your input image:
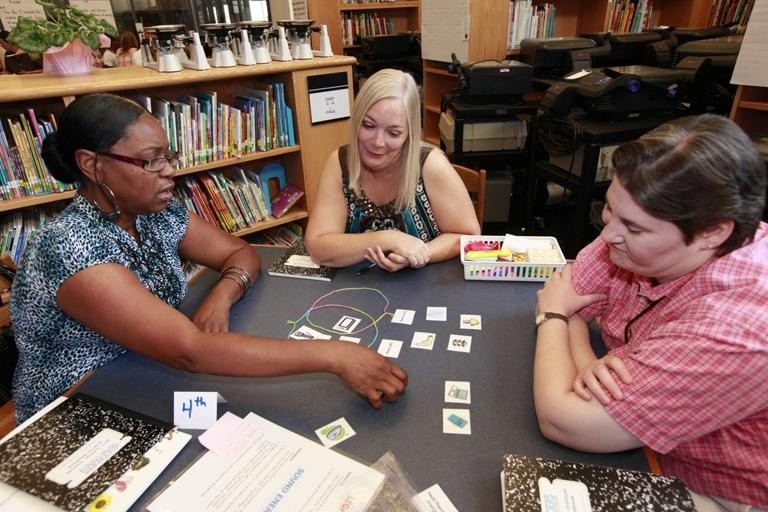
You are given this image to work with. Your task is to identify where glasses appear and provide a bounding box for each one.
[97,150,179,173]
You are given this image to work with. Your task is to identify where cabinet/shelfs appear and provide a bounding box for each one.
[307,0,422,61]
[422,0,768,221]
[0,54,358,330]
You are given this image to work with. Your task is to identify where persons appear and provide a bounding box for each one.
[116,30,140,66]
[0,31,27,71]
[533,112,768,512]
[91,48,119,69]
[304,67,481,272]
[8,92,409,426]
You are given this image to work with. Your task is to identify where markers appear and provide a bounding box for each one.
[464,242,512,259]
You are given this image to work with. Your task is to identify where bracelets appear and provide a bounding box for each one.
[222,264,254,303]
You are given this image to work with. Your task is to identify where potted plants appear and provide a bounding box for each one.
[6,0,119,76]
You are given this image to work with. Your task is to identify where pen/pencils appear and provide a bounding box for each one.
[357,264,376,276]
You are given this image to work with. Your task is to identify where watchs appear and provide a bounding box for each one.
[533,312,570,336]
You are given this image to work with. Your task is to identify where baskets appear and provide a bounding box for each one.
[460,234,567,281]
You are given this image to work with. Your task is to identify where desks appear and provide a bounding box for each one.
[0,244,651,512]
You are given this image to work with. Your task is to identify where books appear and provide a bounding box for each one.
[339,0,396,46]
[0,80,81,311]
[499,452,698,512]
[708,0,755,27]
[506,0,557,51]
[608,0,654,33]
[0,394,177,511]
[137,76,305,246]
